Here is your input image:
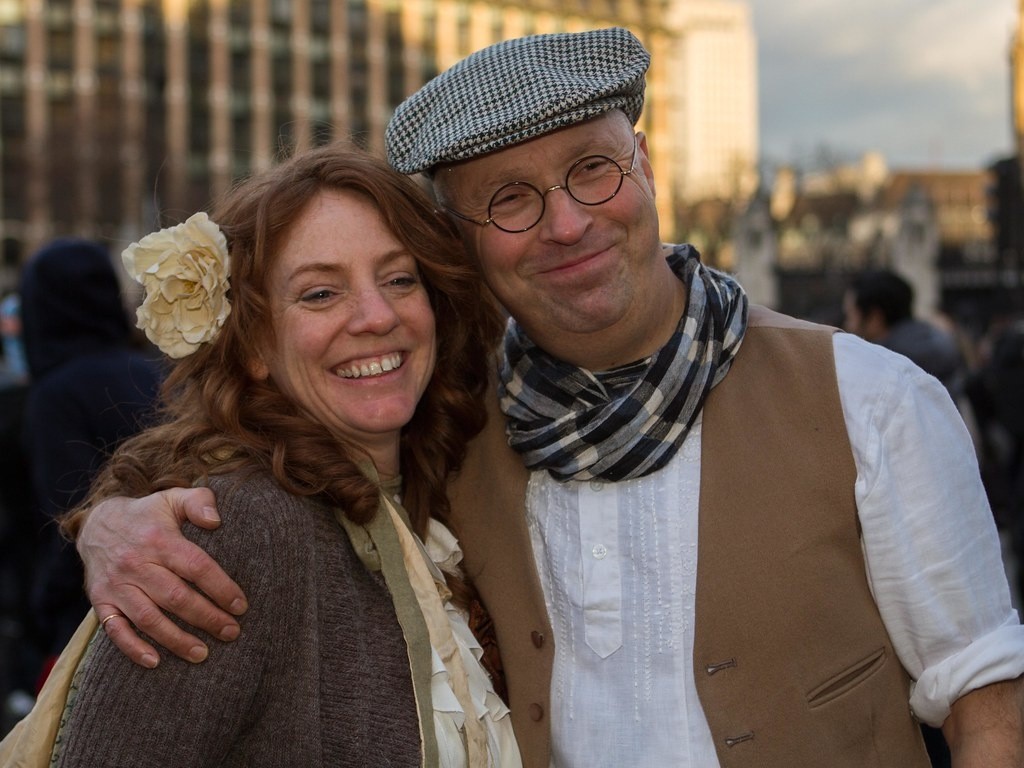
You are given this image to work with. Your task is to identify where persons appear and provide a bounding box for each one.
[47,146,524,768]
[759,156,1023,581]
[74,26,1024,768]
[0,239,180,743]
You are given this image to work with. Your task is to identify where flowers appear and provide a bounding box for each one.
[121,212,232,360]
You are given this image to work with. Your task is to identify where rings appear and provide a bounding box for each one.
[102,614,125,631]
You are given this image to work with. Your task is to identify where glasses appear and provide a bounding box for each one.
[441,135,638,234]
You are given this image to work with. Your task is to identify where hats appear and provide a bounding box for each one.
[385,26,649,176]
